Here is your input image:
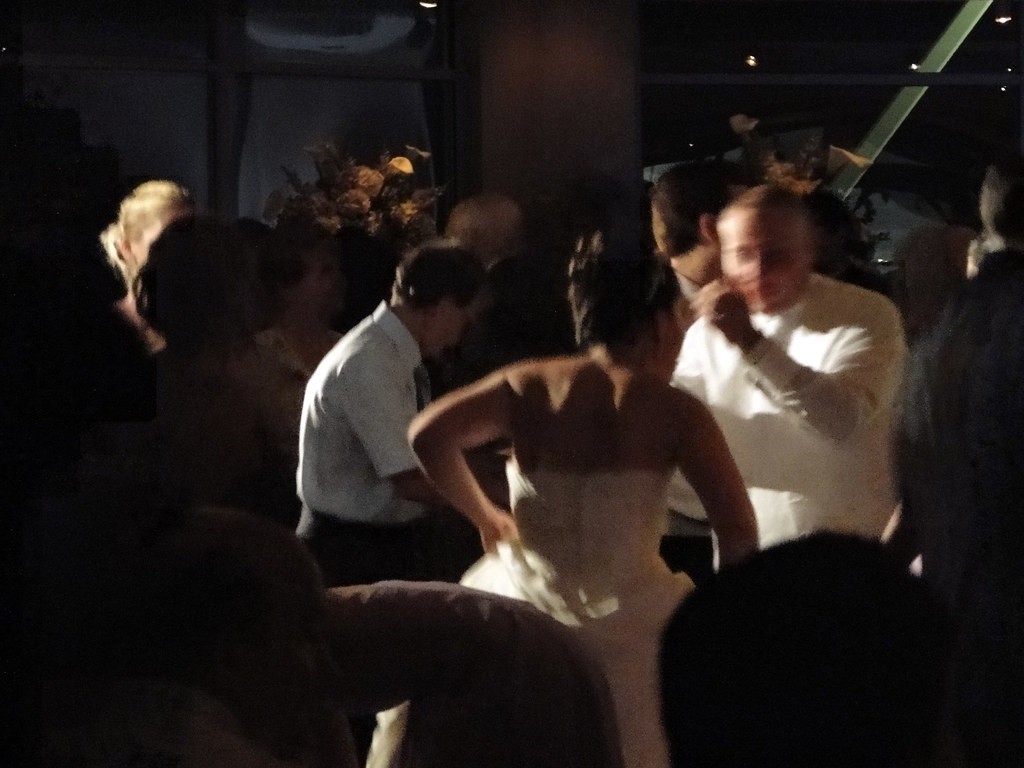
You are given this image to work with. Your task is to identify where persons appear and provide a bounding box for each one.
[0,115,1024,768]
